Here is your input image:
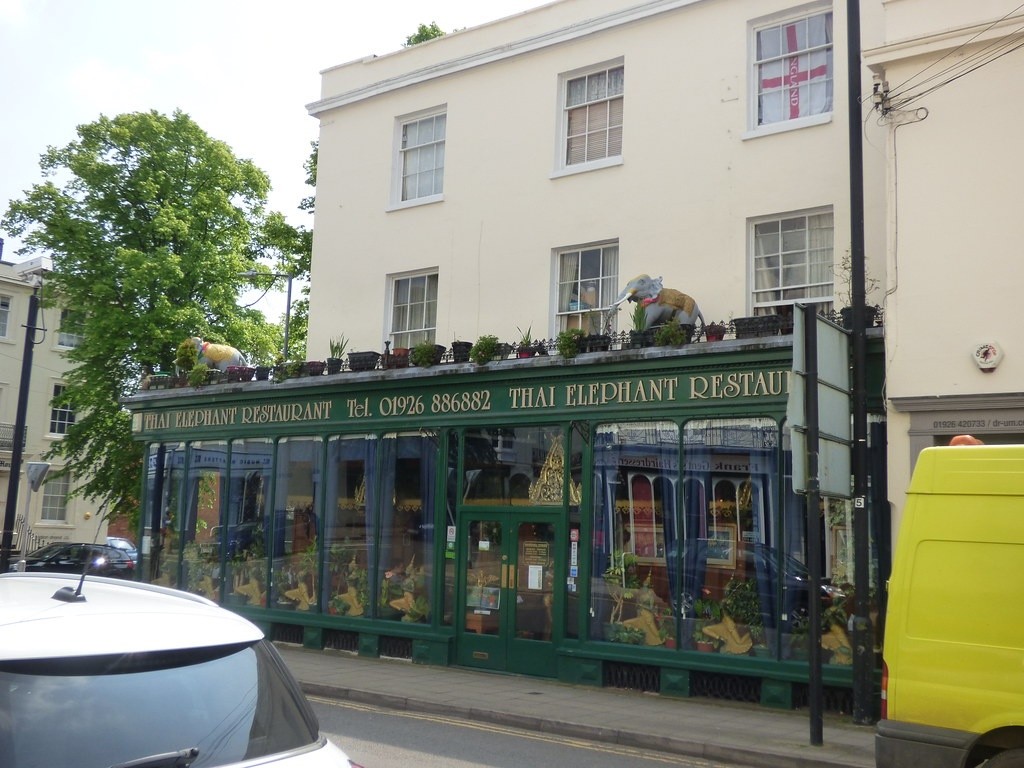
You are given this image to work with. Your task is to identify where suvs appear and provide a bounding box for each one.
[8,541,137,582]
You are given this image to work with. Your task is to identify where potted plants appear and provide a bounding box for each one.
[271,356,309,384]
[647,316,696,348]
[514,321,537,359]
[701,320,728,343]
[160,501,855,663]
[409,341,447,369]
[627,303,655,348]
[830,242,880,331]
[326,333,349,377]
[471,335,512,367]
[585,306,623,352]
[186,363,224,391]
[556,328,586,360]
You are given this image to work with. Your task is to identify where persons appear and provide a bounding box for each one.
[384,560,405,588]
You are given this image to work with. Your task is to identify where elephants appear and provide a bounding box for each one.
[604,274,705,340]
[174,337,247,372]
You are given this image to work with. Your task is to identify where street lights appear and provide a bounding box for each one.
[237,270,295,364]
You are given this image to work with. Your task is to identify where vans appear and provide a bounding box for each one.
[873,433,1024,768]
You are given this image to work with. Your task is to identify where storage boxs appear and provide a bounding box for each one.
[569,301,591,311]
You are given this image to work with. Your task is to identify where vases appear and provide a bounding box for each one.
[309,361,326,378]
[346,351,382,372]
[226,365,269,381]
[392,348,410,369]
[451,341,473,364]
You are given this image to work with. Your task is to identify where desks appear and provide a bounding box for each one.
[467,612,498,635]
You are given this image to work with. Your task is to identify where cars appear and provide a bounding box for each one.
[104,537,139,566]
[0,567,358,768]
[625,538,855,633]
